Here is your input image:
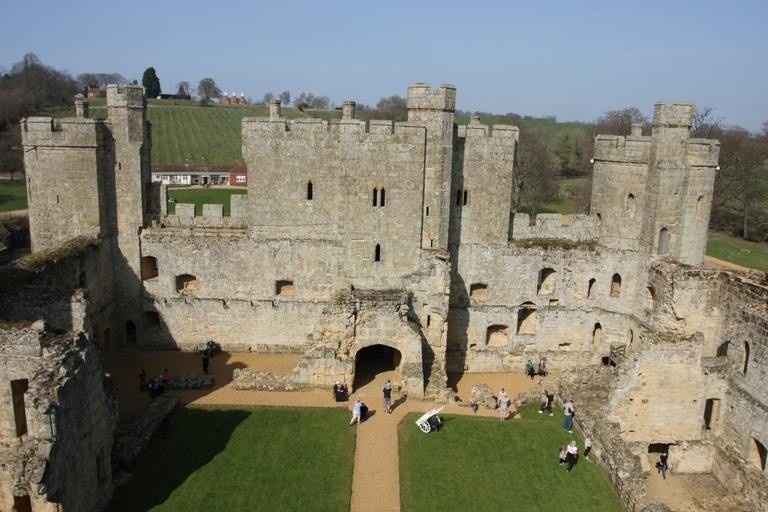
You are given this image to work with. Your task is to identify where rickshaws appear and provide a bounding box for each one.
[414,405,446,434]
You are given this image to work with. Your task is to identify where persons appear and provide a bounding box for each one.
[528,367,535,382]
[161,368,172,382]
[583,434,592,460]
[527,360,534,375]
[562,399,576,433]
[659,451,668,480]
[498,394,509,423]
[333,381,343,393]
[538,359,546,377]
[497,387,505,407]
[538,390,553,416]
[384,379,391,390]
[139,368,146,393]
[147,376,156,397]
[565,440,578,472]
[382,383,392,413]
[559,445,567,465]
[156,380,166,396]
[348,398,361,426]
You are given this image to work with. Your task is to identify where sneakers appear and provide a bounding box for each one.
[537,410,554,417]
